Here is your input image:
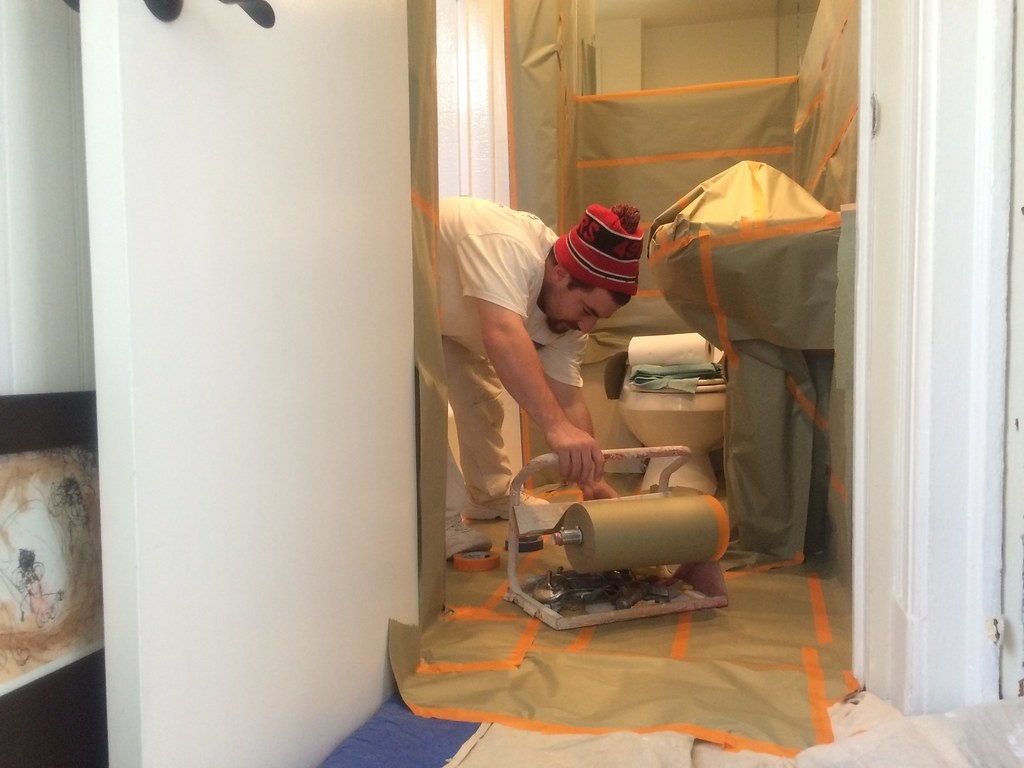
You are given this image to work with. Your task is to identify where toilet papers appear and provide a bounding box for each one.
[627,331,715,368]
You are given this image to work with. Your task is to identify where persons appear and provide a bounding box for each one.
[438,195,644,563]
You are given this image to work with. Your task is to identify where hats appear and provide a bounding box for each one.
[553,204,645,296]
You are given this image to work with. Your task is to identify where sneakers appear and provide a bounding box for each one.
[463,487,549,519]
[445,515,492,559]
